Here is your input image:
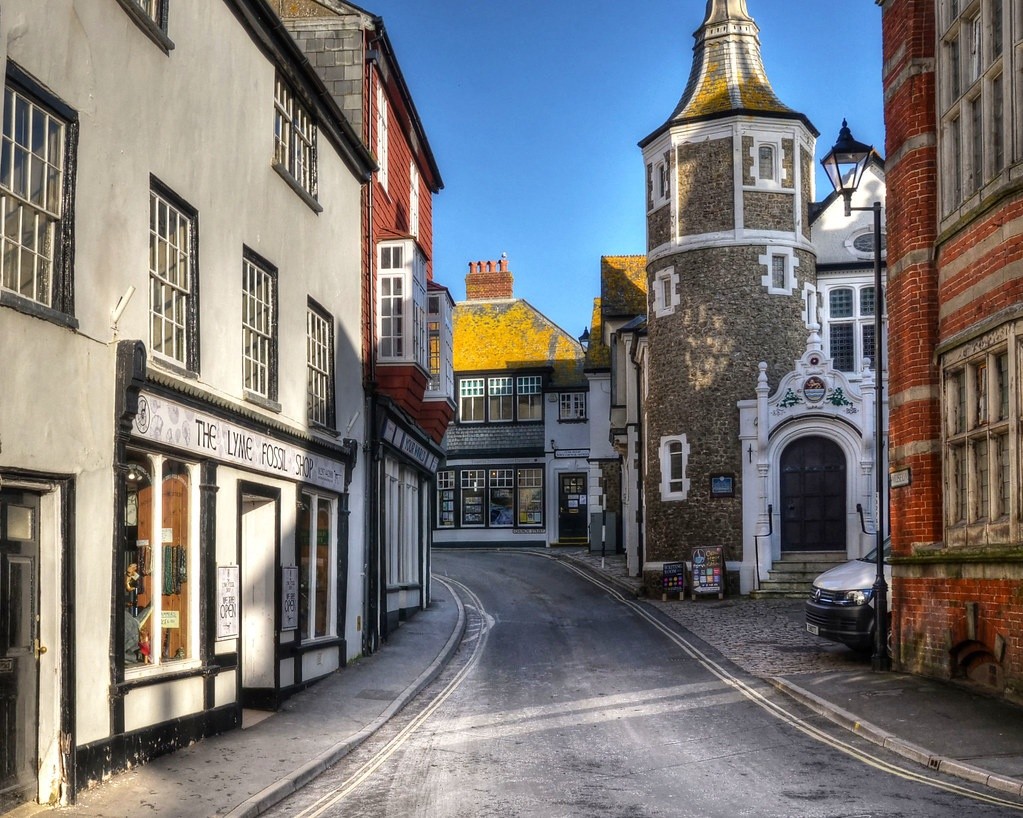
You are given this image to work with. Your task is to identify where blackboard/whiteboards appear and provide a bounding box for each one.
[690,545,725,595]
[662,562,684,593]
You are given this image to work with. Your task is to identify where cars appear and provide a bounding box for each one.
[805,534,892,651]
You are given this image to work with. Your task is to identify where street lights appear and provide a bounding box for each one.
[819,116,888,671]
